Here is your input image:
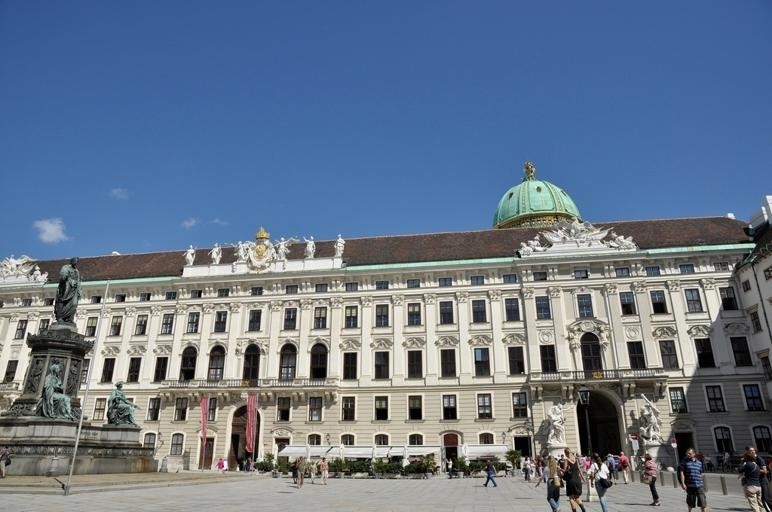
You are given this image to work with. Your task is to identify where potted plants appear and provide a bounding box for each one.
[454,449,522,479]
[253,452,440,479]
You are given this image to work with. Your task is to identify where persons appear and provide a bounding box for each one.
[481,459,497,487]
[307,460,318,484]
[292,461,298,483]
[733,450,766,512]
[742,445,772,512]
[181,233,346,267]
[0,445,11,478]
[557,446,588,512]
[584,452,611,512]
[677,447,708,512]
[545,403,566,445]
[638,400,658,442]
[215,458,224,473]
[106,380,142,428]
[520,450,634,486]
[693,447,772,482]
[446,457,453,479]
[296,455,306,488]
[52,255,81,325]
[534,454,563,512]
[643,453,661,506]
[39,363,76,420]
[319,457,330,485]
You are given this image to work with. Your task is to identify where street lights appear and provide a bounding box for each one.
[524,418,532,461]
[575,381,594,459]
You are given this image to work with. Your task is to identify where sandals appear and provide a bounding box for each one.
[653,501,660,506]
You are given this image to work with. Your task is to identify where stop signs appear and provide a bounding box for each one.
[631,432,637,441]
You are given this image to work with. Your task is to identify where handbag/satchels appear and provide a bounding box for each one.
[643,476,652,484]
[554,476,565,488]
[623,460,628,468]
[600,478,612,489]
[563,466,573,480]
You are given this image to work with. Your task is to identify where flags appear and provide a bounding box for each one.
[199,393,207,448]
[245,392,256,453]
